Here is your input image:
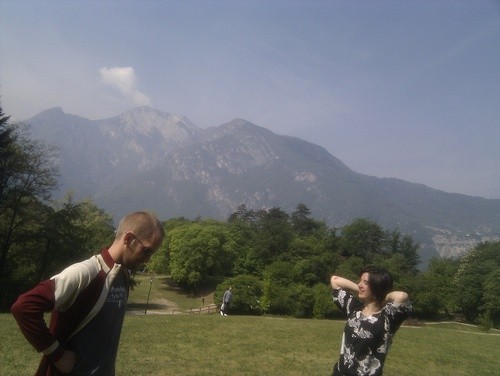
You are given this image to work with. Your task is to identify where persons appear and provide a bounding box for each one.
[330,267,412,376]
[11,211,166,376]
[220,286,233,317]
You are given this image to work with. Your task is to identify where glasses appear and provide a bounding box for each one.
[134,236,155,257]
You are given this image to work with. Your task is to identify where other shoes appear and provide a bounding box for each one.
[219,311,223,316]
[224,313,228,317]
